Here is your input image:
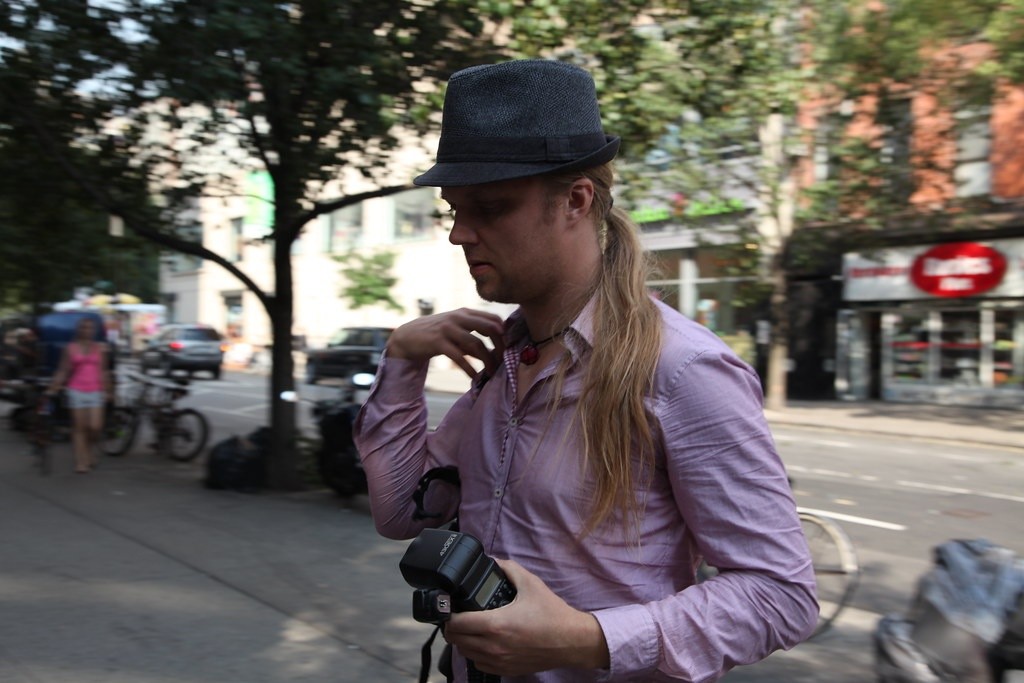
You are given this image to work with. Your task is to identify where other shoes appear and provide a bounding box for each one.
[76,457,89,472]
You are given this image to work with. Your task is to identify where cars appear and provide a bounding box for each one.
[139,324,227,384]
[303,326,393,385]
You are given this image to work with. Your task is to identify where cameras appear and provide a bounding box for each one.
[400,528,517,626]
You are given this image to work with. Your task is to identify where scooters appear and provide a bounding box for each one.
[871,535,1024,682]
[280,371,377,504]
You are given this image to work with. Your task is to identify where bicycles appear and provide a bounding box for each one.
[101,373,212,463]
[786,473,857,644]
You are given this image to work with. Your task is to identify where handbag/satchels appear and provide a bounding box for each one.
[51,386,69,406]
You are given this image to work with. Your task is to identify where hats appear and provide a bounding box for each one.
[412,59,621,186]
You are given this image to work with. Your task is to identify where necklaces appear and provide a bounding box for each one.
[518,324,569,365]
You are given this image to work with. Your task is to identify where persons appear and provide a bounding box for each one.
[353,59,820,682]
[45,316,114,474]
